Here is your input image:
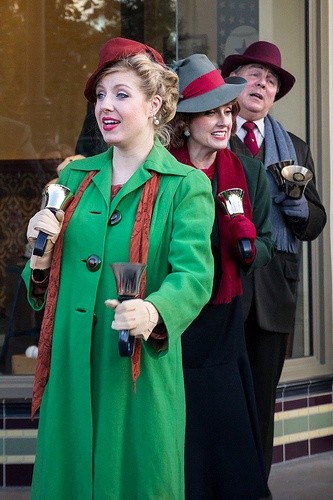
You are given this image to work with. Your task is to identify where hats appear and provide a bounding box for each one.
[84,37,163,103]
[171,53,247,126]
[221,41,296,103]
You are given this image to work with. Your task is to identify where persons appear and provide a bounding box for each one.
[21,37,216,500]
[220,41,328,500]
[167,54,276,500]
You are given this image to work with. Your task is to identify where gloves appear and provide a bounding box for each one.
[274,191,309,224]
[105,298,158,342]
[26,209,65,269]
[225,214,256,264]
